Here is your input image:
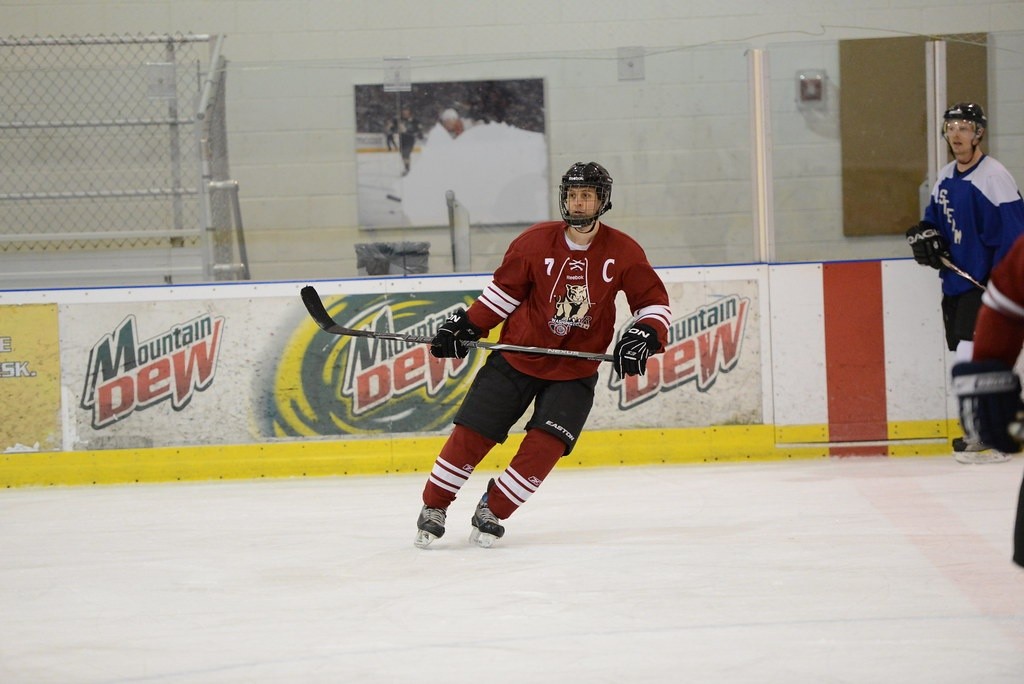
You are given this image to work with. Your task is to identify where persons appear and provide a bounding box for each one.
[412,161,672,547]
[950,231,1024,567]
[385,119,398,151]
[905,102,1024,465]
[395,104,422,175]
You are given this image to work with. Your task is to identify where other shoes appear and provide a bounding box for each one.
[402,168,409,176]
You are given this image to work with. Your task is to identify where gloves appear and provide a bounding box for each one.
[431,308,480,359]
[906,221,951,270]
[613,324,662,379]
[952,362,1024,455]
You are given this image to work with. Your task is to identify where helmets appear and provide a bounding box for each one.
[557,162,614,227]
[942,102,985,141]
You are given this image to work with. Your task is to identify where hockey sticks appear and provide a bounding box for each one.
[299,285,614,363]
[940,256,987,289]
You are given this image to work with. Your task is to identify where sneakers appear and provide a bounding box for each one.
[951,437,1011,464]
[469,478,504,548]
[415,504,448,548]
